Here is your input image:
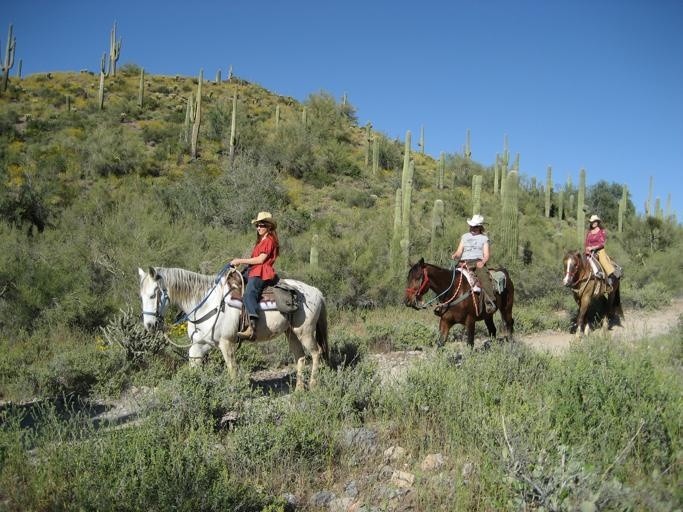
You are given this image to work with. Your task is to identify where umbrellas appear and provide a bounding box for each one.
[610,274,617,283]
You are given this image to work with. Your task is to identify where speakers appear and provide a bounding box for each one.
[237,319,256,341]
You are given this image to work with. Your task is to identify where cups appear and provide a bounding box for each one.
[256,224,266,228]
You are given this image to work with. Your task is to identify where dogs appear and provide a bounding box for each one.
[562,246,626,339]
[403,256,514,354]
[137,266,329,395]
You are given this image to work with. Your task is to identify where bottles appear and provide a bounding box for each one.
[251,212,277,230]
[467,215,484,227]
[589,215,601,222]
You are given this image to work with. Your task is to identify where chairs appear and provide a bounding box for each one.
[486,303,497,312]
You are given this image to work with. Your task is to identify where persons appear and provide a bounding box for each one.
[450,215,496,314]
[584,214,617,280]
[229,211,280,341]
